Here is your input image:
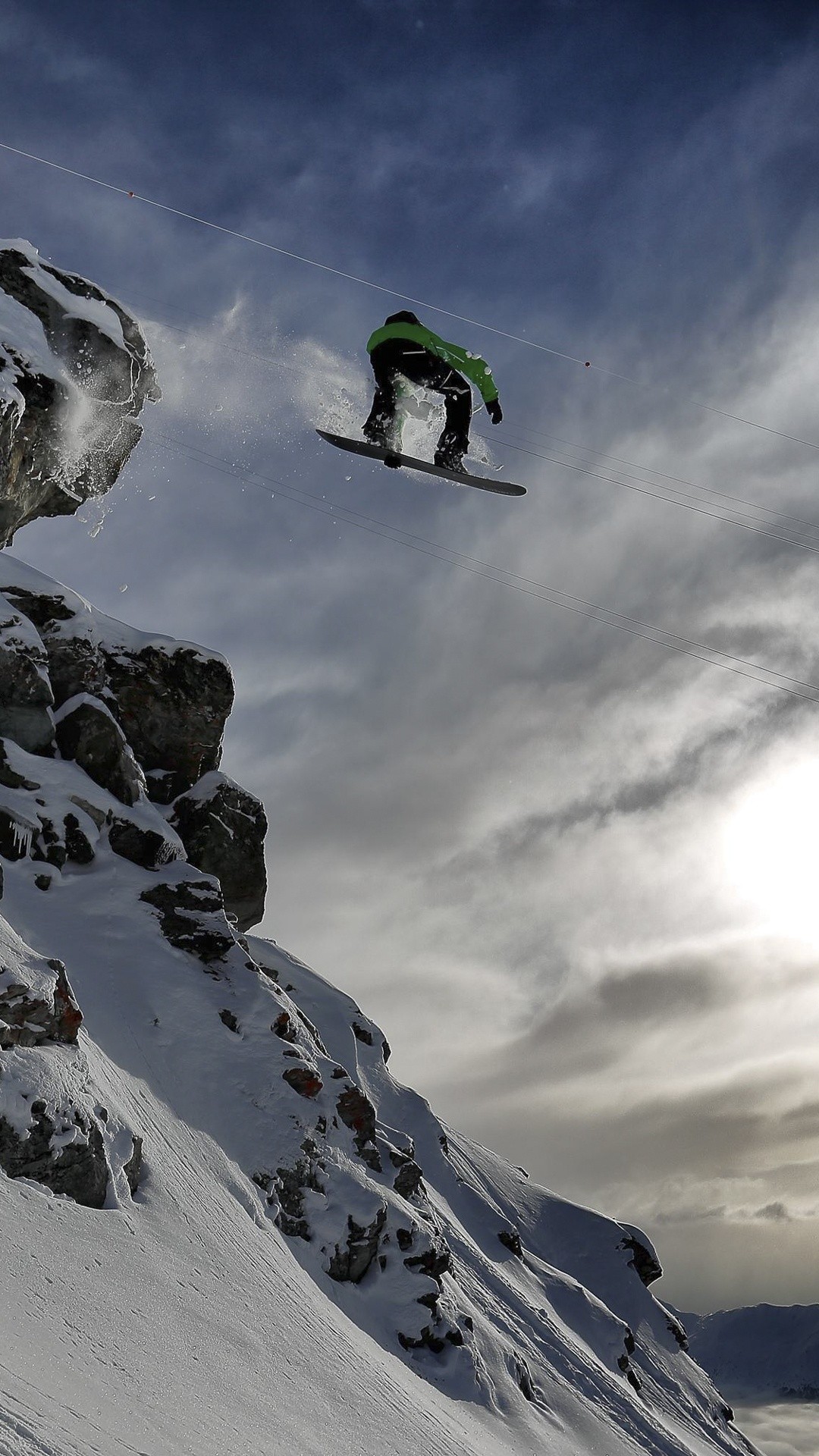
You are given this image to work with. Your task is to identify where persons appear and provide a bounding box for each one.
[362,311,504,477]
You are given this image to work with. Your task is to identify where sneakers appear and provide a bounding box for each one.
[368,434,401,454]
[434,451,467,474]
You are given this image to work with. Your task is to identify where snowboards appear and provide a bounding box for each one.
[315,428,527,496]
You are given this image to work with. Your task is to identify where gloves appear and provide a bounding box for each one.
[486,398,503,426]
[384,455,402,469]
